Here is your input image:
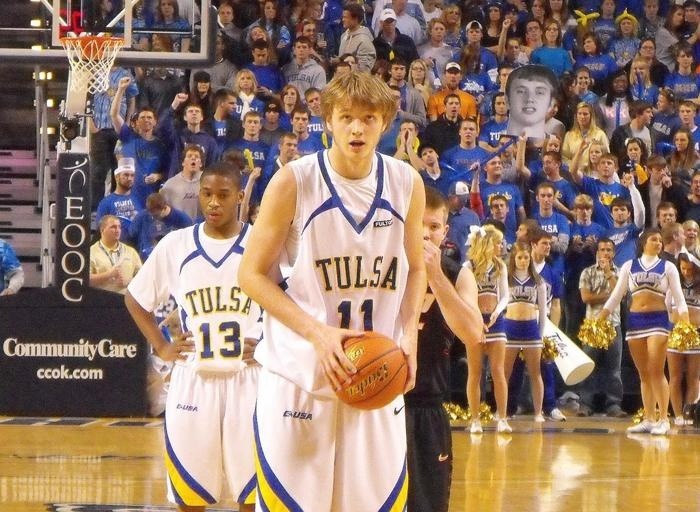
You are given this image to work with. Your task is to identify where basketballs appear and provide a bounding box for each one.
[332,332,408,411]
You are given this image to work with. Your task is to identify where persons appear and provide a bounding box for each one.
[46,2,700,434]
[126,70,481,511]
[0,239,25,295]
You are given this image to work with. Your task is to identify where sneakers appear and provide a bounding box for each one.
[498,420,512,433]
[470,420,482,432]
[576,410,588,416]
[544,409,566,422]
[607,409,626,417]
[628,413,692,433]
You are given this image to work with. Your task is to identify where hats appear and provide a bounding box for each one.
[445,63,461,72]
[379,9,395,21]
[467,19,483,29]
